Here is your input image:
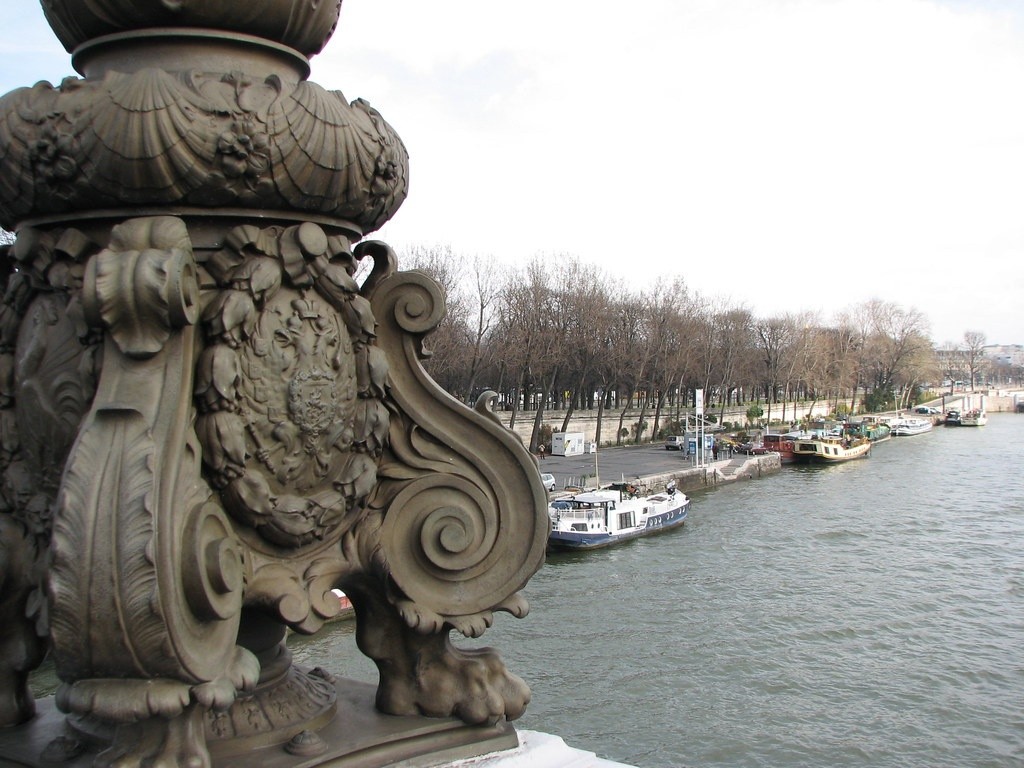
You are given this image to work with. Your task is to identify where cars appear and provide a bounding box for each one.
[539,473,556,492]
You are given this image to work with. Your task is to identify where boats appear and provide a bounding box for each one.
[860,407,988,444]
[546,446,692,552]
[791,425,872,463]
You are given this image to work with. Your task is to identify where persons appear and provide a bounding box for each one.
[745,423,748,433]
[837,412,848,421]
[728,442,733,458]
[712,443,719,460]
[538,443,545,459]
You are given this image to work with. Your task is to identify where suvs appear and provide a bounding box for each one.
[665,435,684,451]
